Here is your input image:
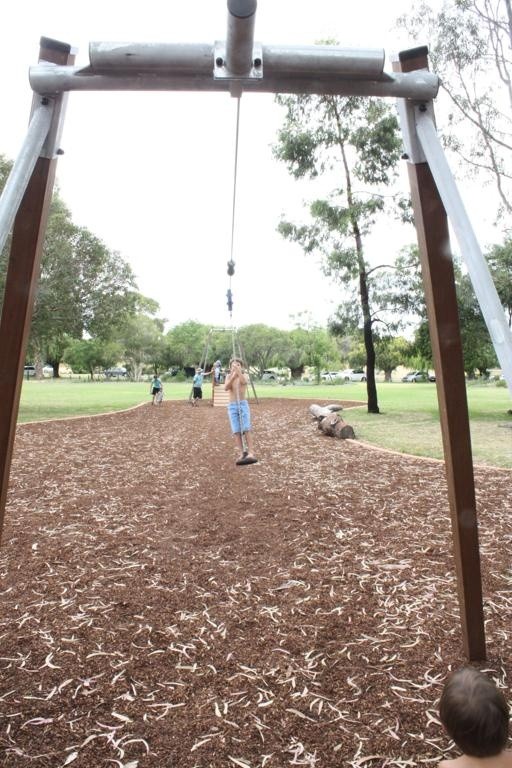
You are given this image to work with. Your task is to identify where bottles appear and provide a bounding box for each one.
[196,368,203,372]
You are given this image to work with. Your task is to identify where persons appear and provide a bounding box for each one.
[191,367,215,406]
[214,362,221,386]
[155,388,165,406]
[150,374,163,404]
[224,359,253,459]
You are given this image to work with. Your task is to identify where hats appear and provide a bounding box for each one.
[22,365,35,376]
[168,366,180,375]
[261,366,436,382]
[41,364,53,374]
[102,365,129,377]
